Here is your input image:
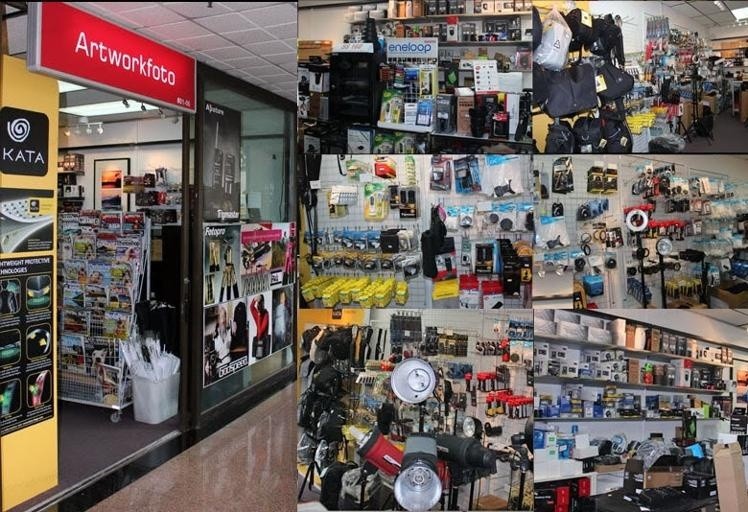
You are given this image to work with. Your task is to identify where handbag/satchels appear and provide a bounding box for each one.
[598,62,636,101]
[531,59,600,118]
[598,95,625,123]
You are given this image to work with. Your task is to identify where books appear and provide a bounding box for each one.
[58,211,152,405]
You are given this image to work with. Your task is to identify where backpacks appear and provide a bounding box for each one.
[573,112,604,154]
[604,114,635,154]
[544,119,575,154]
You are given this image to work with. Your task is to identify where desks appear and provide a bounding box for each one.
[299,115,533,155]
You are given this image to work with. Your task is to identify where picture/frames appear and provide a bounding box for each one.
[729,357,748,415]
[93,157,130,214]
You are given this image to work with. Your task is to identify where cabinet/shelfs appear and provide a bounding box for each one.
[534,333,736,422]
[58,212,151,422]
[345,11,533,74]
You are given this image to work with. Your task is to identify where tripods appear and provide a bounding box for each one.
[674,79,715,145]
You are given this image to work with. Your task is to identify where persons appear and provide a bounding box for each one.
[274,289,293,342]
[249,292,270,340]
[212,303,239,364]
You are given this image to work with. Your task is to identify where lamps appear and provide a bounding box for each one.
[390,358,443,511]
[656,239,673,308]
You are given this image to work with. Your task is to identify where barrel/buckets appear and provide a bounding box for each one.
[131,371,180,425]
